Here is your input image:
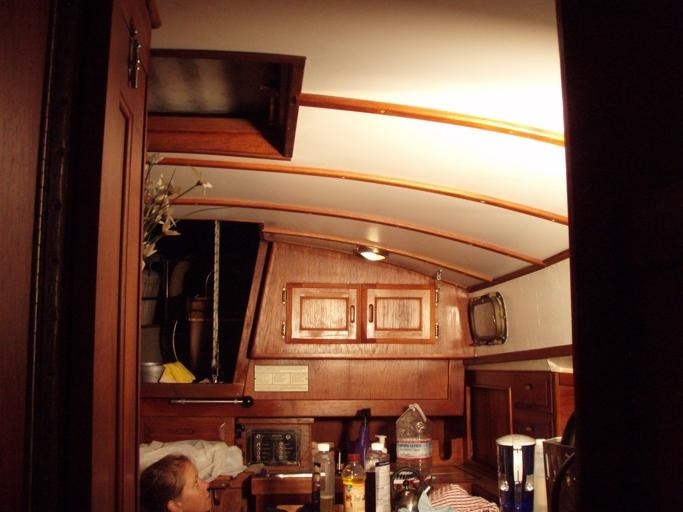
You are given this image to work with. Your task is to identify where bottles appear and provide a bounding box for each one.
[310,440,336,512]
[340,452,367,512]
[364,441,385,475]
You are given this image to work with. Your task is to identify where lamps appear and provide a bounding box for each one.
[356,245,389,262]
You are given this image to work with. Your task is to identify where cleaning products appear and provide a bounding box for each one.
[353,407,370,464]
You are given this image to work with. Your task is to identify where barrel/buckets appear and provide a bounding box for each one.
[396,403,433,478]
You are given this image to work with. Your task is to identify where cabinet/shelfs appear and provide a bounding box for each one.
[281,282,439,345]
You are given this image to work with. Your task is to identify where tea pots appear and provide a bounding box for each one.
[390,467,433,512]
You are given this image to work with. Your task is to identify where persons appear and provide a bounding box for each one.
[140,453,212,512]
